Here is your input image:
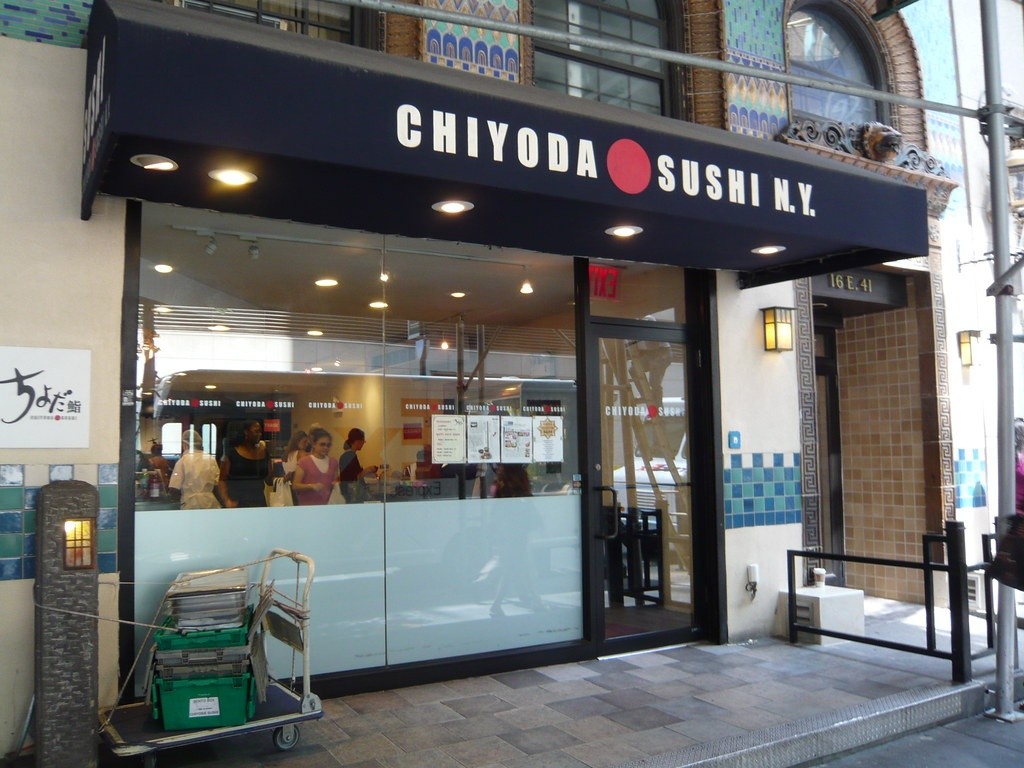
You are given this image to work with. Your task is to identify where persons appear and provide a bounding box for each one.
[339,428,378,504]
[282,431,311,480]
[292,427,338,505]
[148,444,169,493]
[490,463,543,613]
[218,417,292,508]
[168,430,223,510]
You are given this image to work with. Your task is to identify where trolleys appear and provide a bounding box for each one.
[94,547,325,768]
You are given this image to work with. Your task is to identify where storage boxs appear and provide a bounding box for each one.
[144,567,257,732]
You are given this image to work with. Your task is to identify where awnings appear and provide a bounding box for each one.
[80,0,929,291]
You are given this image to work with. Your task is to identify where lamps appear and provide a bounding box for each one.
[956,330,981,367]
[130,155,178,172]
[745,563,760,601]
[208,169,258,185]
[431,200,474,214]
[604,225,643,237]
[63,516,94,570]
[759,305,798,353]
[751,245,786,255]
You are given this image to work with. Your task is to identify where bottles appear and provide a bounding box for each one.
[149,471,160,497]
[141,469,149,492]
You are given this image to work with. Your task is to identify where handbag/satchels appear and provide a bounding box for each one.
[270,477,293,507]
[327,481,346,505]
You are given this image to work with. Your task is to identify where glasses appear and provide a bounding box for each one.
[316,442,332,447]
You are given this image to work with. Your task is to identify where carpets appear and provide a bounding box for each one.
[605,622,649,638]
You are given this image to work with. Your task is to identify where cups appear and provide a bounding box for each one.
[813,568,826,587]
[401,481,412,486]
[412,481,423,487]
